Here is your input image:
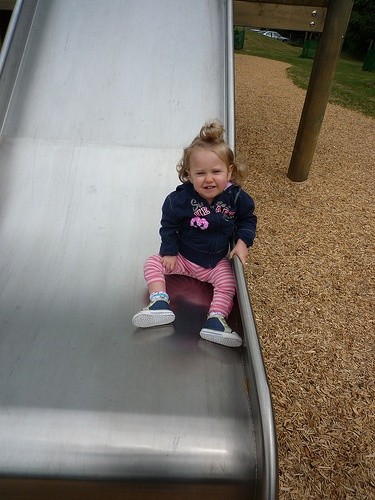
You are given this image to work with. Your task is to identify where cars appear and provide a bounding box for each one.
[263,31,290,43]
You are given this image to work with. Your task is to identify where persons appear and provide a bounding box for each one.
[131,119,258,348]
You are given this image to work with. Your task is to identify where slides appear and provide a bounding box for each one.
[1,0,282,500]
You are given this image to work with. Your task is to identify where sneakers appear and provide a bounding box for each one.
[200,314,242,347]
[132,298,175,327]
